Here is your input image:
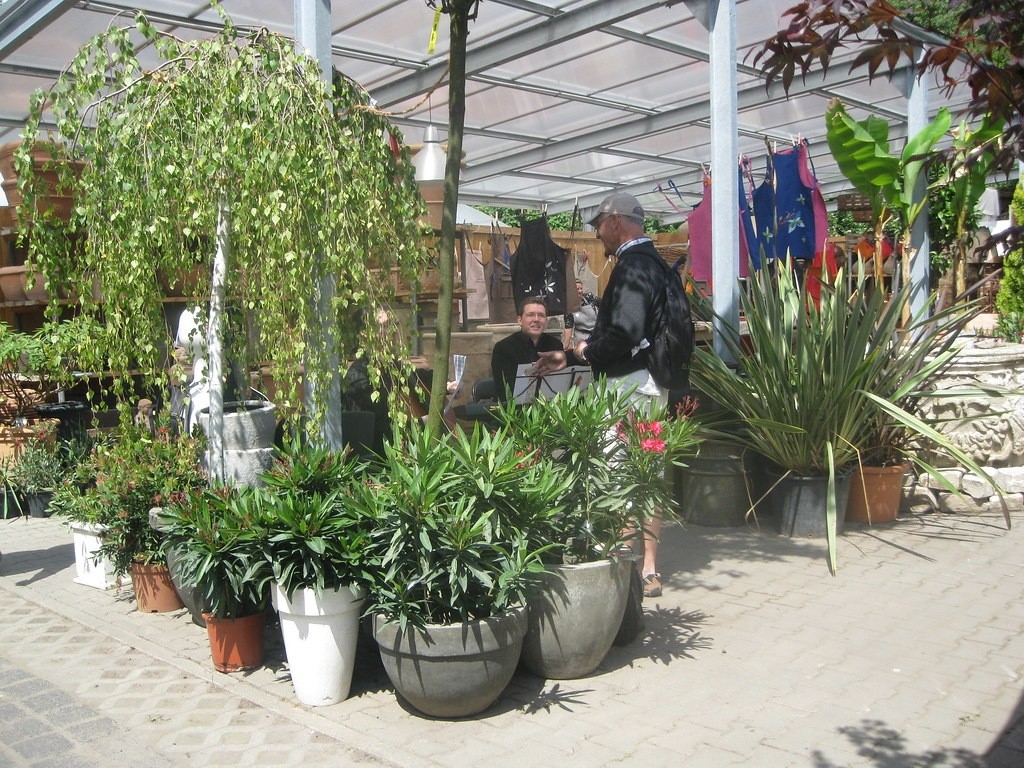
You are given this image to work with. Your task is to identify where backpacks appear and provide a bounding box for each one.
[627,245,696,391]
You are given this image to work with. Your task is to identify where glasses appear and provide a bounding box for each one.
[525,312,545,318]
[595,214,613,230]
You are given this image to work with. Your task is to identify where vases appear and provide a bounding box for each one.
[196,398,276,450]
[66,521,133,590]
[149,506,278,629]
[23,489,56,516]
[270,580,367,708]
[372,599,529,718]
[520,544,632,680]
[201,607,266,673]
[128,561,185,614]
[0,139,209,300]
[0,485,23,518]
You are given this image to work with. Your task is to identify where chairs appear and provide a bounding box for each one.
[473,379,499,403]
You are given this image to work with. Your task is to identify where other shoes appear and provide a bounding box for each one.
[643,572,662,597]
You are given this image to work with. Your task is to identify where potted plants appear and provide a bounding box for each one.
[683,239,1013,577]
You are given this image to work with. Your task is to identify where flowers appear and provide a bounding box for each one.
[0,365,707,637]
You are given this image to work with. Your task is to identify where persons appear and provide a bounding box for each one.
[525,193,695,600]
[343,310,463,438]
[134,399,155,433]
[170,348,195,387]
[562,278,602,350]
[492,296,564,401]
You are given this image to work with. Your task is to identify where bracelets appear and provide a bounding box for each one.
[581,346,587,365]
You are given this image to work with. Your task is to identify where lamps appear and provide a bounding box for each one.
[411,89,468,185]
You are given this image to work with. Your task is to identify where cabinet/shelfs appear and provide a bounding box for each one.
[368,228,476,357]
